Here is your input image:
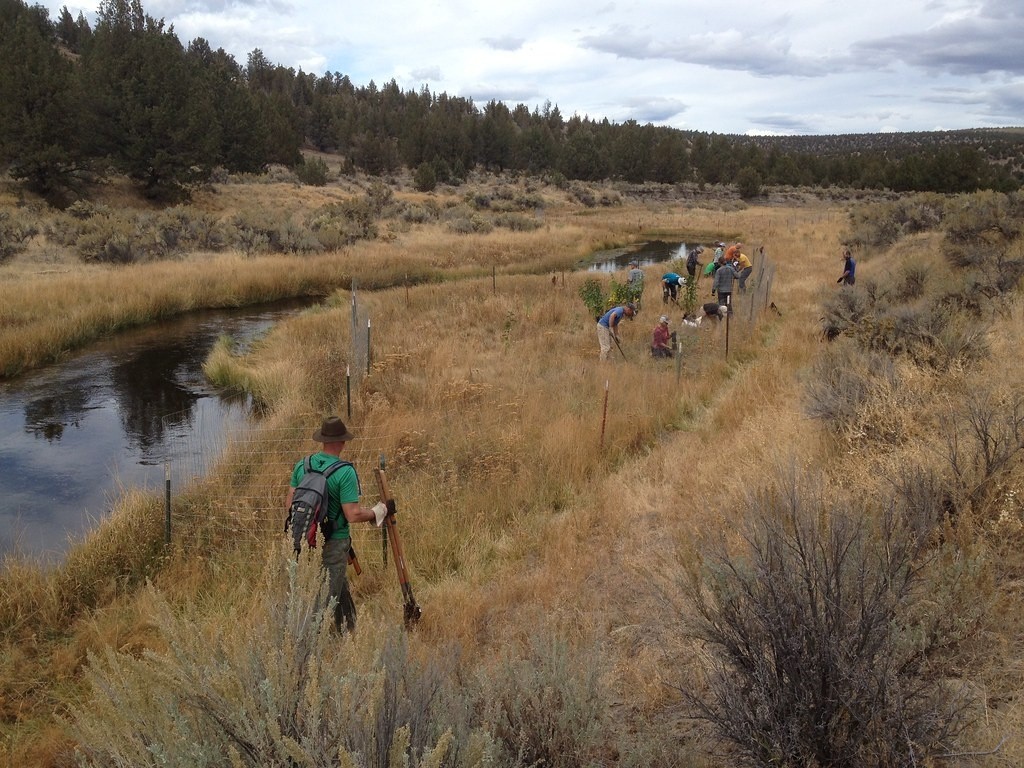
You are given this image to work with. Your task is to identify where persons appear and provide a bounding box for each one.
[626,260,644,309]
[704,240,725,277]
[597,303,635,359]
[724,244,741,264]
[651,317,672,357]
[712,257,735,313]
[733,250,752,288]
[682,303,727,327]
[686,246,704,276]
[836,250,856,286]
[286,417,388,630]
[660,272,687,302]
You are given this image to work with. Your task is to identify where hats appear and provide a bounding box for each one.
[678,278,686,286]
[626,302,637,316]
[720,242,725,247]
[312,416,355,443]
[737,243,743,248]
[660,315,672,324]
[720,306,727,316]
[629,261,638,266]
[697,246,704,253]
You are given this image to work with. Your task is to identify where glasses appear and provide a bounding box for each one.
[663,321,668,325]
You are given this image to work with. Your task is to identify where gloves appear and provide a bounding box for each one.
[733,262,739,266]
[369,501,387,527]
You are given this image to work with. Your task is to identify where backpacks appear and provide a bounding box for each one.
[284,454,361,558]
